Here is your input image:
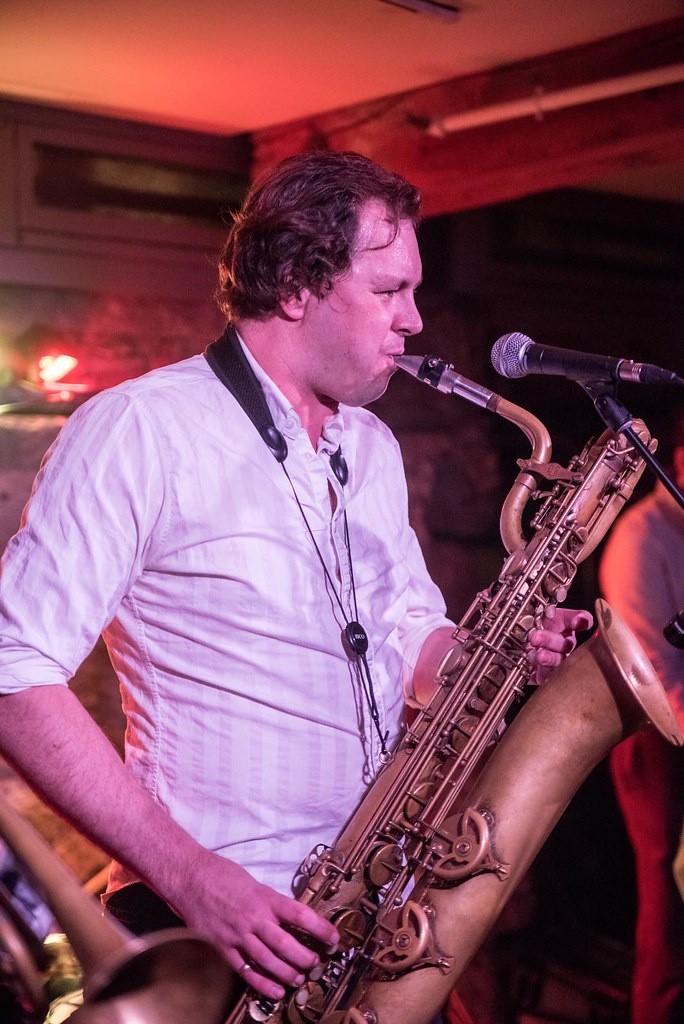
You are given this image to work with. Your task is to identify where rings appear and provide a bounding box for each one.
[239,960,255,974]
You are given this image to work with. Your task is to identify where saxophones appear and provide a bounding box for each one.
[225,354,683,1024]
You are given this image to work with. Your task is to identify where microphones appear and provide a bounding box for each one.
[490,333,684,390]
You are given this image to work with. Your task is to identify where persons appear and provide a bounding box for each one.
[2,135,593,998]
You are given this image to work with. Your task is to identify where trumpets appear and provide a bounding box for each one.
[0,795,223,1023]
